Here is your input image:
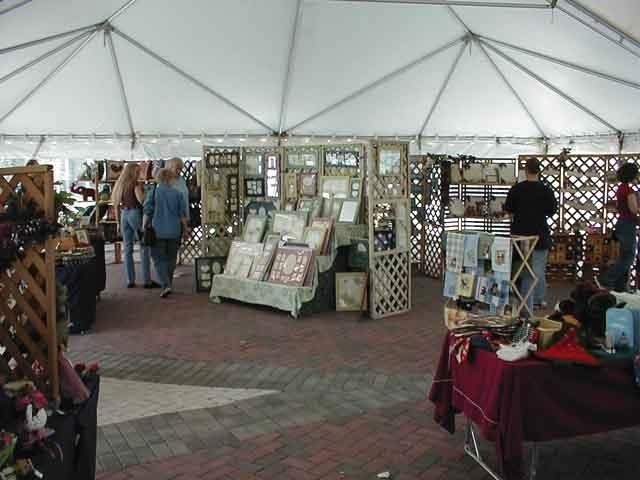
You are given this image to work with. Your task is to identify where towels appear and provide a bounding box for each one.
[442,231,512,312]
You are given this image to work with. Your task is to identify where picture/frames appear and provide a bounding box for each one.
[194,148,411,312]
[75,228,89,244]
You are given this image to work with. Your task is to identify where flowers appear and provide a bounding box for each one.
[0,385,63,480]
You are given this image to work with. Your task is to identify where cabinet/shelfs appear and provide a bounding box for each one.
[95,160,165,244]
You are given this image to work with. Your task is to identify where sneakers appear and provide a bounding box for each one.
[143,280,161,288]
[160,288,172,297]
[128,283,136,287]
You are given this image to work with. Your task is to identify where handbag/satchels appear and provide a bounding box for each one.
[142,222,156,246]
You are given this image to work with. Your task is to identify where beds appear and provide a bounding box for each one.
[103,161,165,243]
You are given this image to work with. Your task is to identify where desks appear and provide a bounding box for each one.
[427,315,638,479]
[56,255,97,320]
[46,376,99,480]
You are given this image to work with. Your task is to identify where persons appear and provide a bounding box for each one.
[23,159,57,220]
[504,158,557,314]
[111,156,192,297]
[608,163,639,292]
